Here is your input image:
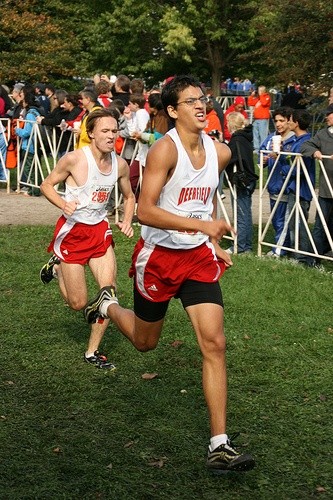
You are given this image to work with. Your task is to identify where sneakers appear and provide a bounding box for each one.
[82,286,117,325]
[39,254,61,285]
[206,432,256,471]
[83,350,117,372]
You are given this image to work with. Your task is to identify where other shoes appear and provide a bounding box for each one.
[266,250,275,255]
[253,149,259,154]
[224,247,233,254]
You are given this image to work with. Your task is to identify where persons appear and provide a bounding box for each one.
[39,106,136,372]
[0,71,333,265]
[270,110,316,261]
[223,111,259,255]
[257,108,295,259]
[84,74,254,471]
[247,84,272,152]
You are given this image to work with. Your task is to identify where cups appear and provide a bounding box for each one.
[272,135,282,152]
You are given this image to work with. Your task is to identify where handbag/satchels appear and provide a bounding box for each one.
[231,169,259,196]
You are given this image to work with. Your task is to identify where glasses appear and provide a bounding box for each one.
[176,96,210,105]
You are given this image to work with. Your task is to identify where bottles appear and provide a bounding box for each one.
[60,119,65,131]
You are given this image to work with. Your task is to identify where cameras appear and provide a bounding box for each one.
[208,130,219,139]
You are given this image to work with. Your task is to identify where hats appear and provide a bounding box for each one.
[321,103,333,114]
